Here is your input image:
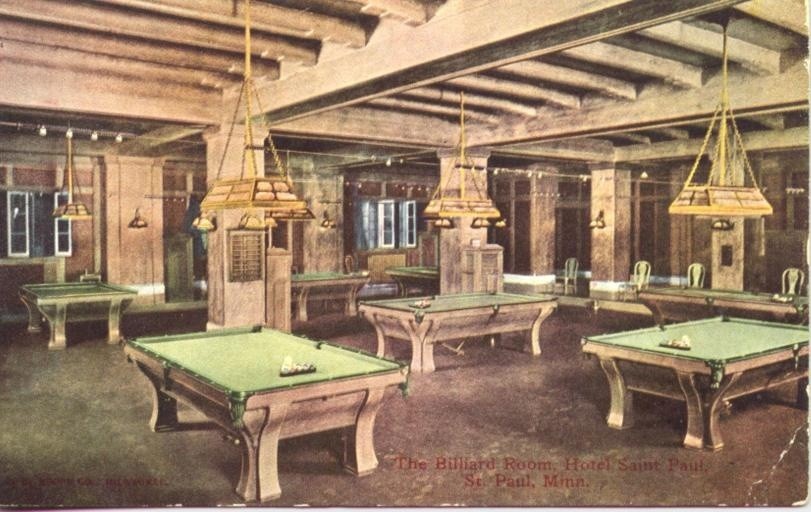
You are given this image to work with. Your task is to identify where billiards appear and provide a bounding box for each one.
[278,354,316,377]
[410,300,431,308]
[773,293,794,302]
[660,335,691,348]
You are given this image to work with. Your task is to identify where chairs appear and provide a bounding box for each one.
[618,261,651,303]
[781,268,804,296]
[686,262,704,288]
[552,258,578,295]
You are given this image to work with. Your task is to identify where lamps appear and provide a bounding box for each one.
[52,128,94,220]
[587,212,604,228]
[191,1,307,230]
[420,87,505,228]
[270,152,315,222]
[668,18,774,215]
[126,209,147,227]
